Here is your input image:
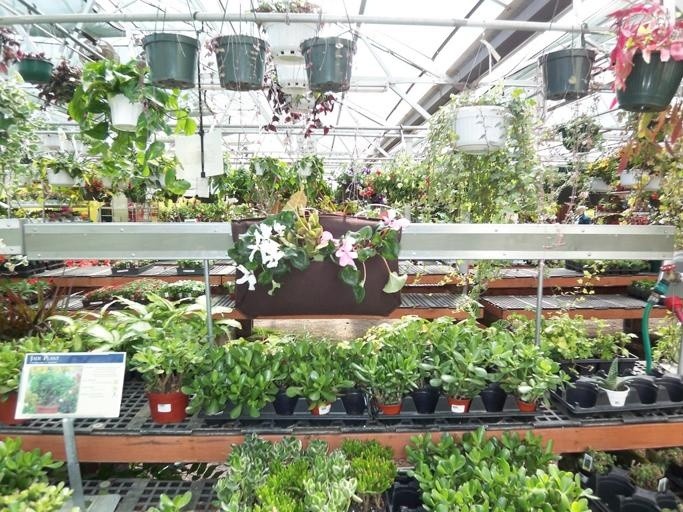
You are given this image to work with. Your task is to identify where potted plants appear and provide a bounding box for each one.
[425,82,561,224]
[554,113,604,153]
[536,44,603,120]
[66,58,196,196]
[29,369,76,413]
[127,1,357,139]
[0,336,37,425]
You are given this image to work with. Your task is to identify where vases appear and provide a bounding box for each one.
[616,46,682,114]
[15,58,53,84]
[580,457,683,510]
[80,292,195,310]
[385,468,423,512]
[29,259,63,274]
[564,259,641,275]
[233,318,253,339]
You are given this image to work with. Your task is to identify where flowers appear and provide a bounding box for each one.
[607,0,683,111]
[82,278,205,300]
[12,50,54,62]
